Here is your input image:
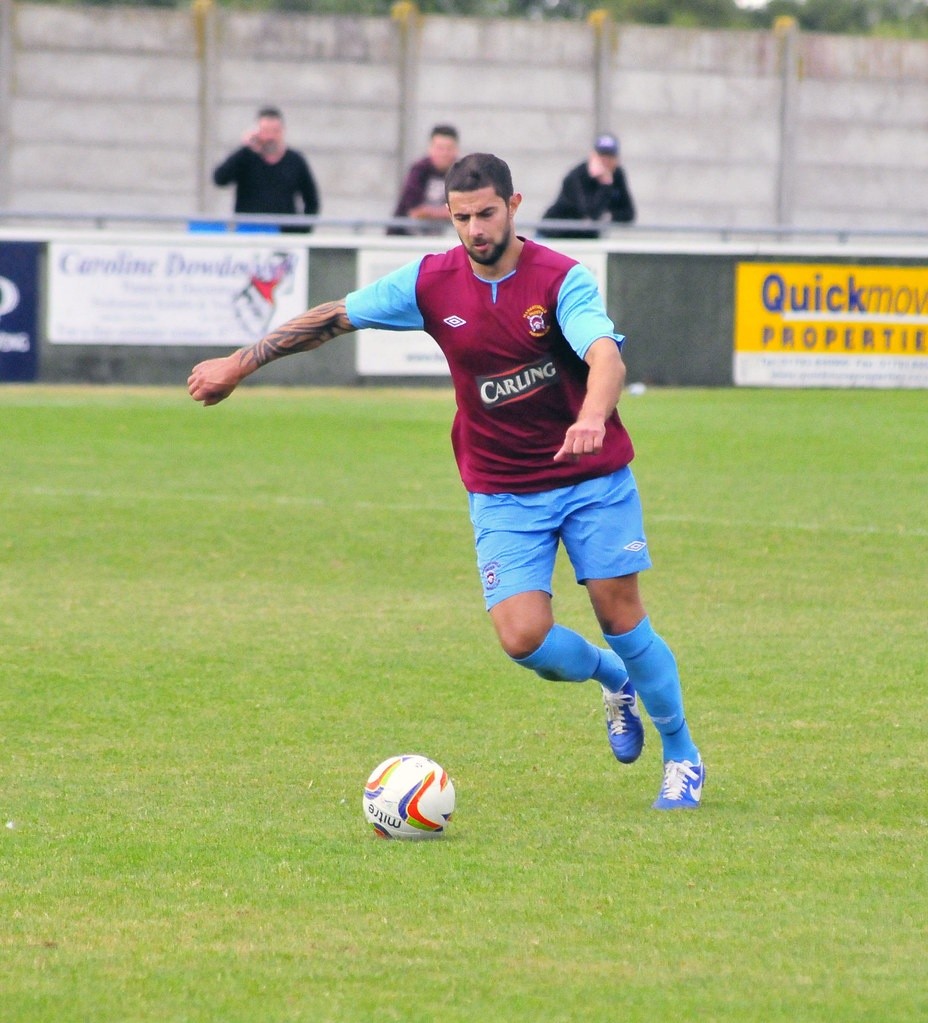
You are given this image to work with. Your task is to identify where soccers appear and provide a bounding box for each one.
[362,754,457,839]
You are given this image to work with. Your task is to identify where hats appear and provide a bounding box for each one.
[593,134,619,156]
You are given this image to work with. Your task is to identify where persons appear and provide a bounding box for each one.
[383,126,460,237]
[535,130,637,240]
[212,107,322,235]
[187,152,708,809]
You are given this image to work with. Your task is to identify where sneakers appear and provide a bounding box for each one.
[601,678,645,763]
[653,752,705,809]
